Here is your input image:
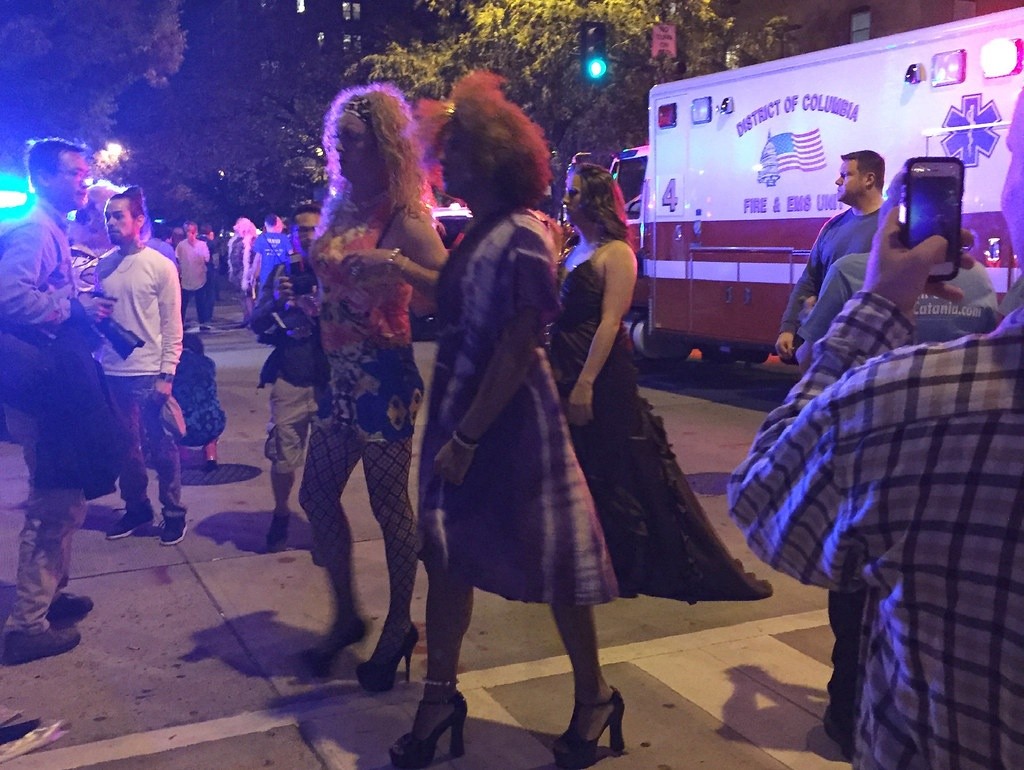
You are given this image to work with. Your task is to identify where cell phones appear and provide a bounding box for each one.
[904,157,965,282]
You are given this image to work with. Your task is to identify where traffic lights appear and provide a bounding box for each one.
[580,21,607,88]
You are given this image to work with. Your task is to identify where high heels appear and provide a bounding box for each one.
[301,619,366,678]
[553,685,625,770]
[355,620,419,692]
[388,692,468,770]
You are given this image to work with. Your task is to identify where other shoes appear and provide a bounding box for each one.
[199,321,212,331]
[267,510,290,550]
[824,705,856,763]
[204,458,219,473]
[237,317,250,329]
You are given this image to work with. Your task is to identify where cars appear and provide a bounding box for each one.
[407,207,477,328]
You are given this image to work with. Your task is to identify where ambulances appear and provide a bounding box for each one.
[608,5,1024,371]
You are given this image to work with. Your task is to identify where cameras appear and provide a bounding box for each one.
[91,291,146,360]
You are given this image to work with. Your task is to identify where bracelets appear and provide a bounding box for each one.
[452,429,479,449]
[387,248,401,275]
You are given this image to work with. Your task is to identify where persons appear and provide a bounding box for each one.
[795,173,1001,759]
[390,76,626,770]
[0,140,114,664]
[299,89,450,692]
[775,150,885,358]
[548,164,773,601]
[727,93,1024,770]
[143,206,335,543]
[78,186,187,545]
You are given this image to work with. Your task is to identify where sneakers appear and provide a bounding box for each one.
[157,516,188,545]
[44,592,94,622]
[1,624,81,665]
[106,504,155,539]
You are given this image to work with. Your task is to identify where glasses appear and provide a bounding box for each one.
[51,169,93,183]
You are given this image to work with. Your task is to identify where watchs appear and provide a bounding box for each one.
[159,372,174,382]
